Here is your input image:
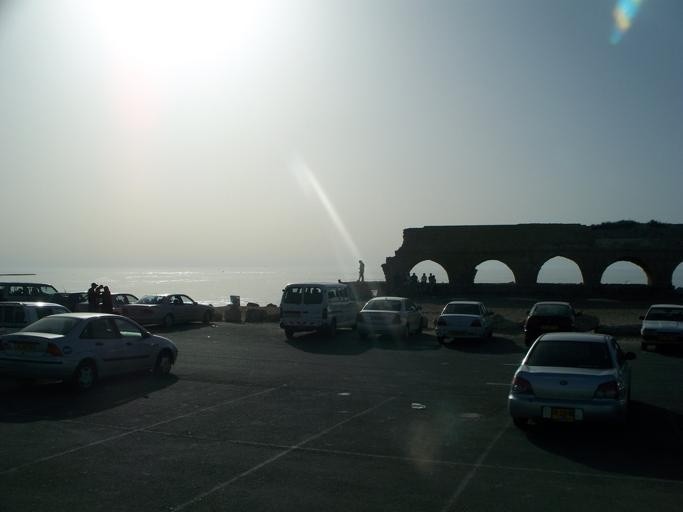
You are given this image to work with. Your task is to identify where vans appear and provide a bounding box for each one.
[278,281,361,338]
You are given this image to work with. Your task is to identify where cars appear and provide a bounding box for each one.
[506,332,636,427]
[0,282,178,393]
[435,300,494,344]
[638,303,683,350]
[358,296,425,339]
[120,293,214,329]
[523,301,581,346]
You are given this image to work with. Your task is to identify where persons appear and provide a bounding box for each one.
[101,286,113,313]
[410,273,417,283]
[430,276,436,286]
[88,283,98,311]
[421,273,427,284]
[358,260,365,282]
[95,285,104,293]
[429,273,436,284]
